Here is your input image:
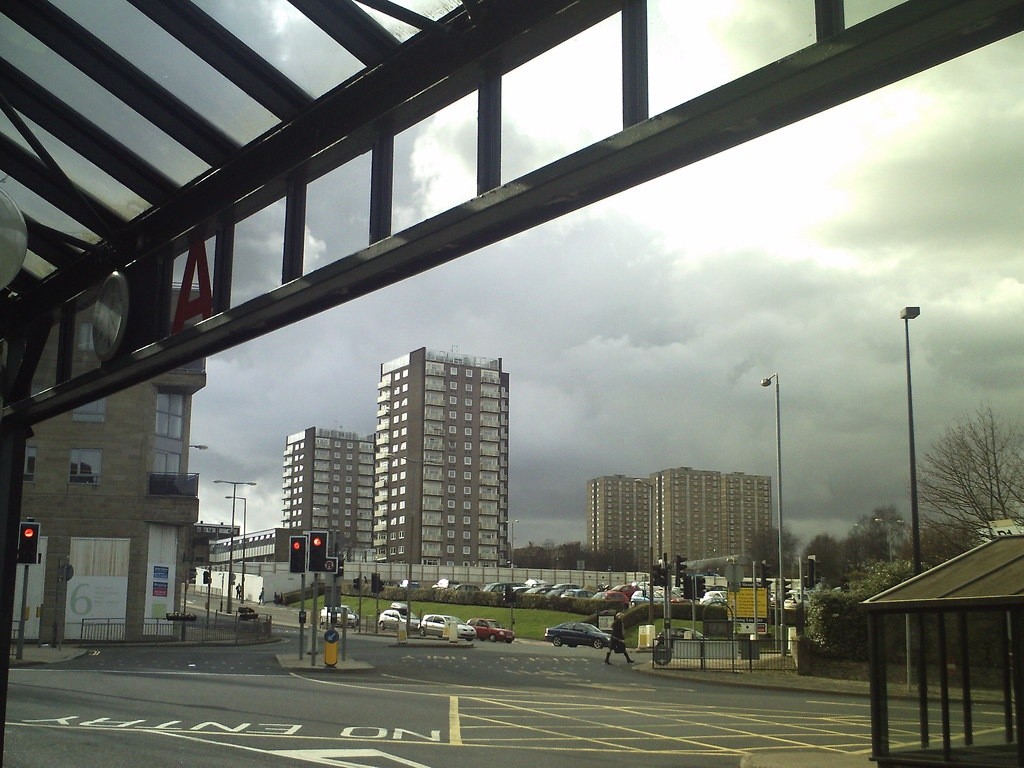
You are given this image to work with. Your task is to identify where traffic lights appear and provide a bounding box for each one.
[681,575,704,601]
[653,563,666,586]
[782,581,792,599]
[18,522,41,564]
[762,563,772,589]
[805,556,821,591]
[676,557,686,584]
[290,532,338,574]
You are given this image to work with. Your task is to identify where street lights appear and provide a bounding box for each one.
[901,306,922,574]
[215,479,257,615]
[637,479,655,627]
[761,373,786,658]
[388,453,441,632]
[504,519,519,580]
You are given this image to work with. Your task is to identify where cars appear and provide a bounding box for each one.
[466,617,615,649]
[377,603,422,630]
[397,578,808,606]
[319,606,361,629]
[418,614,477,642]
[655,627,707,646]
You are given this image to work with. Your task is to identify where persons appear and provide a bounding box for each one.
[364,577,368,584]
[598,583,611,592]
[236,584,242,600]
[274,592,278,605]
[605,612,634,665]
[258,588,265,605]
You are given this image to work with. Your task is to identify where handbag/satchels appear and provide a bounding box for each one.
[614,642,625,654]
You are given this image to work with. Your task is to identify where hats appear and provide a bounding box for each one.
[616,612,624,620]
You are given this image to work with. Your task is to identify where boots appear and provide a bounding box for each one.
[624,653,634,663]
[605,652,612,665]
